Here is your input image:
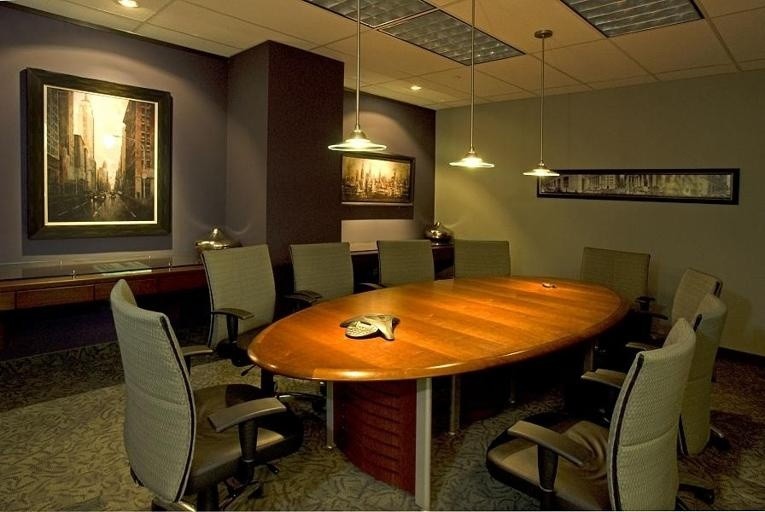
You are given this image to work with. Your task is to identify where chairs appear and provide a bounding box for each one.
[588,293,730,499]
[630,266,724,363]
[485,319,697,511]
[289,241,382,304]
[454,241,511,279]
[108,279,306,510]
[361,238,435,288]
[579,247,655,304]
[200,243,324,433]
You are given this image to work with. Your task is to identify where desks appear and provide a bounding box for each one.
[248,276,633,511]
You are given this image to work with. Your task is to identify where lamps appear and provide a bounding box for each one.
[449,0,495,170]
[522,30,560,178]
[328,2,388,151]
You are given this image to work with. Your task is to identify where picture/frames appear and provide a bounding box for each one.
[21,67,173,246]
[534,167,740,204]
[336,151,416,209]
